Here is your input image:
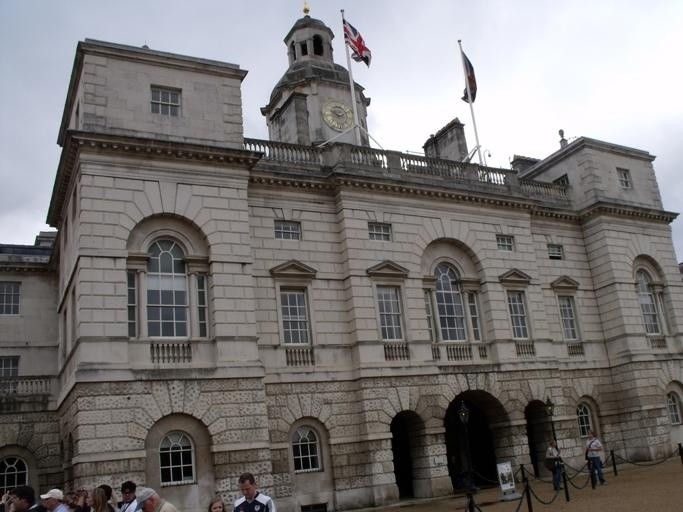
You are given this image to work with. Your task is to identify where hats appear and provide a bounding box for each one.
[134,487,155,512]
[40,488,64,500]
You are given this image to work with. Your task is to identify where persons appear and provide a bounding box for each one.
[0,480,180,512]
[207,497,227,512]
[584,429,609,490]
[545,438,566,491]
[231,472,277,512]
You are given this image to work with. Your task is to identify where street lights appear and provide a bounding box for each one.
[544,395,558,443]
[457,400,477,493]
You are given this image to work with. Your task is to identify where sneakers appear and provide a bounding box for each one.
[554,487,563,490]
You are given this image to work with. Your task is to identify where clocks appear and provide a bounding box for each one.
[323,100,355,133]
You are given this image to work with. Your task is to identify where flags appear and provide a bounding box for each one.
[343,18,371,68]
[460,50,478,104]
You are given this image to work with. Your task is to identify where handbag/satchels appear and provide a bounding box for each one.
[544,458,556,472]
